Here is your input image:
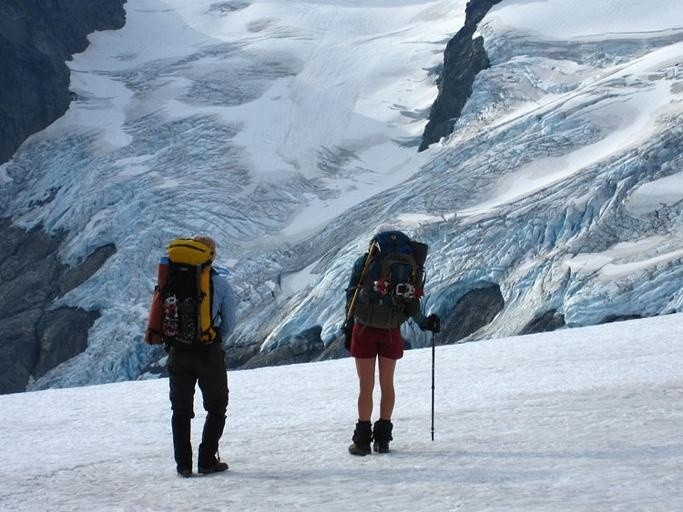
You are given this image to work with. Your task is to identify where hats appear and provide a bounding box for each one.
[192,236,217,262]
[372,223,400,239]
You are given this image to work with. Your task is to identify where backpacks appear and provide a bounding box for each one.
[142,237,222,347]
[345,252,421,323]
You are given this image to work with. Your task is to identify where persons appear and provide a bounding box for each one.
[168,235,234,478]
[345,223,440,456]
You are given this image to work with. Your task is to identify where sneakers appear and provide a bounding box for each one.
[198,461,229,475]
[348,444,372,457]
[177,464,192,478]
[372,443,390,454]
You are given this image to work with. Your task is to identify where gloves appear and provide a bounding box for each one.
[343,326,352,353]
[419,314,441,333]
